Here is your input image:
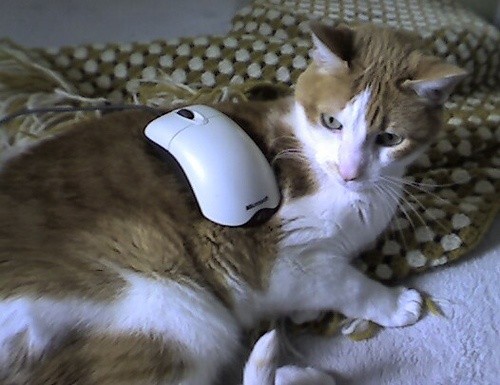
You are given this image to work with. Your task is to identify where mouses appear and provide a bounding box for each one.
[144,104,281,227]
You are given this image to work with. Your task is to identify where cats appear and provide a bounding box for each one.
[0,22,469,384]
